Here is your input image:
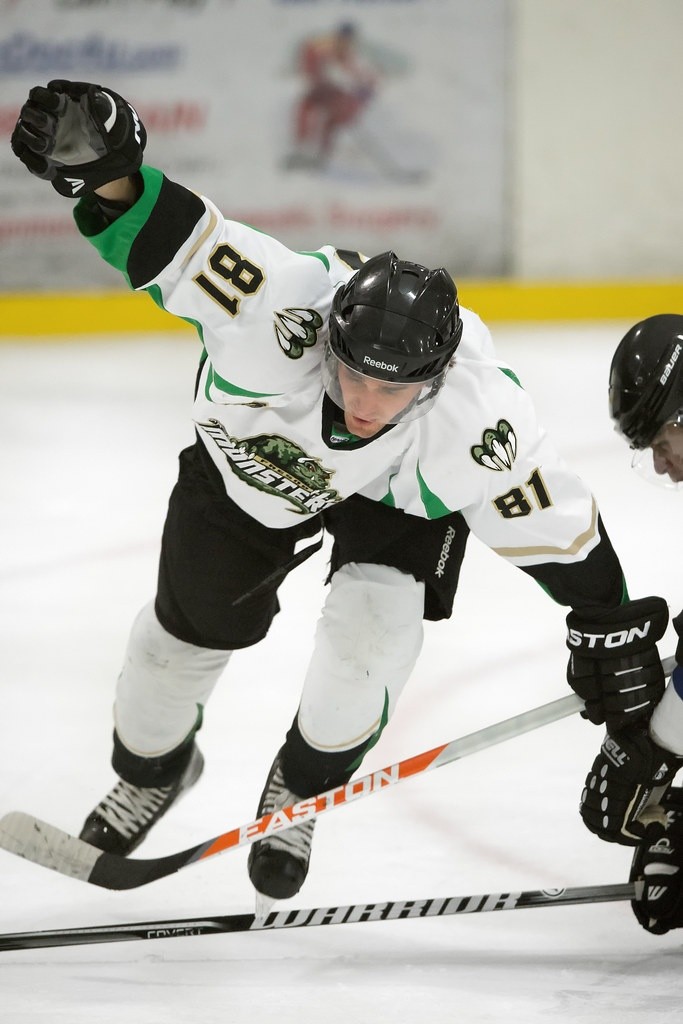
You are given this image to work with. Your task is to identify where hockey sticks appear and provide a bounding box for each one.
[0,652,678,891]
[1,873,646,946]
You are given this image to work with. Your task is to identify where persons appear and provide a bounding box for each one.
[286,23,375,168]
[580,313,683,934]
[12,81,670,909]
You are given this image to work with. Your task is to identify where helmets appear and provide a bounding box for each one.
[327,249,463,384]
[609,314,683,449]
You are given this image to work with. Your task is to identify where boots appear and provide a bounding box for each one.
[78,703,205,857]
[247,708,381,922]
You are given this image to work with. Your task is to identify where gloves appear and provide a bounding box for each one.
[580,728,683,846]
[12,79,148,197]
[566,596,669,735]
[628,788,683,935]
